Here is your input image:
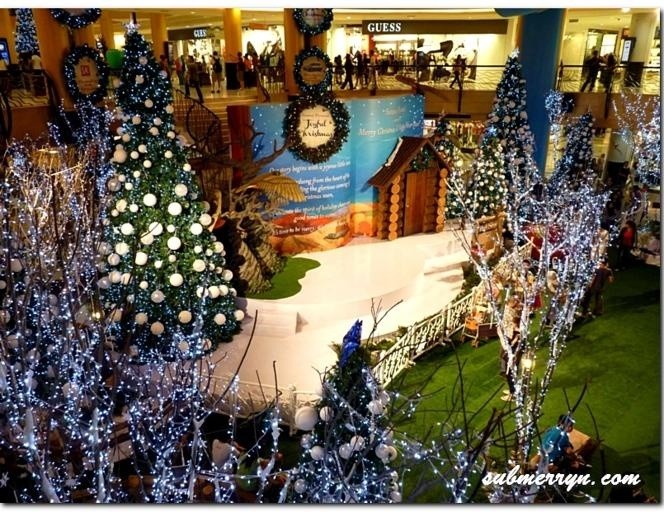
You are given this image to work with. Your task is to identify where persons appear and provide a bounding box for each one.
[212,429,272,494]
[334,50,378,91]
[449,54,462,90]
[539,414,585,499]
[28,48,43,75]
[576,220,638,319]
[579,50,615,93]
[159,51,259,104]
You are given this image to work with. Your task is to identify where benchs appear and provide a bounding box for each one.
[518,429,592,478]
